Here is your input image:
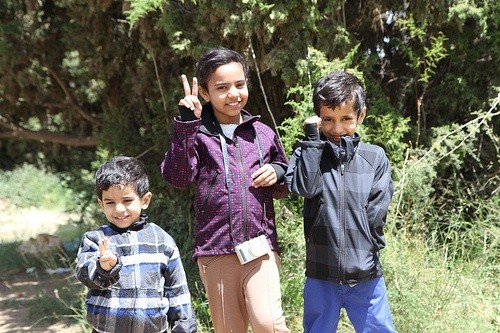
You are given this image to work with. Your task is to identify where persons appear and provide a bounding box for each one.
[159,47,293,332]
[280,68,399,333]
[73,154,200,333]
